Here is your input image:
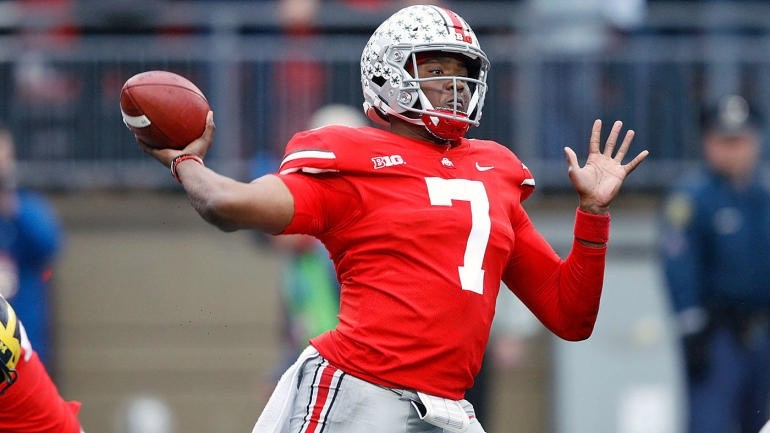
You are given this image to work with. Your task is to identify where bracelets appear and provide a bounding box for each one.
[170,154,204,184]
[574,207,610,243]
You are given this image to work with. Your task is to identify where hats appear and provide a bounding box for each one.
[700,98,761,134]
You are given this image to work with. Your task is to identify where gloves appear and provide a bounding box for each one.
[681,324,714,385]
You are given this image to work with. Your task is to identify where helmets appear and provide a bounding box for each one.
[359,5,491,140]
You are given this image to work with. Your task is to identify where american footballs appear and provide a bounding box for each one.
[120,70,210,150]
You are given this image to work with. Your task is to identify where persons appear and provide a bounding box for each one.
[657,93,770,433]
[0,129,86,433]
[136,6,649,433]
[513,0,648,160]
[257,101,367,352]
